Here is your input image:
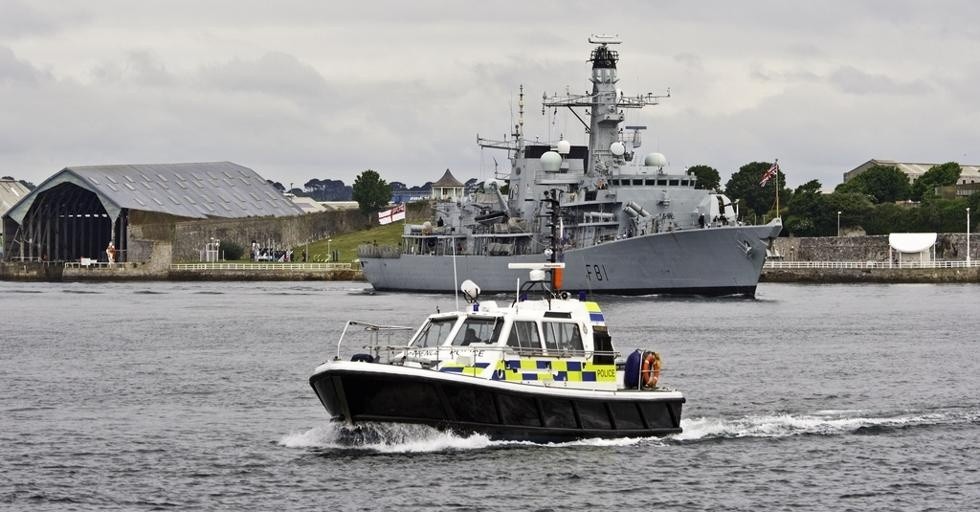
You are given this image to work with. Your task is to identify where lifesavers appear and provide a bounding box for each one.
[644,354,660,387]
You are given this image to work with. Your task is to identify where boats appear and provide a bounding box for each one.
[308,187,686,444]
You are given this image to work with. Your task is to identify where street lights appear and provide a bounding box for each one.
[838,212,841,236]
[966,208,971,260]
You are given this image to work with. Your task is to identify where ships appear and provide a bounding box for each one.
[357,31,784,302]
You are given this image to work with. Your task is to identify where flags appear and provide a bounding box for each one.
[759,162,776,188]
[377,203,404,226]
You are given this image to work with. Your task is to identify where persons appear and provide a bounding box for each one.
[437,217,443,227]
[713,216,720,222]
[106,242,115,268]
[720,213,728,225]
[699,213,705,228]
[460,329,481,346]
[260,248,306,263]
